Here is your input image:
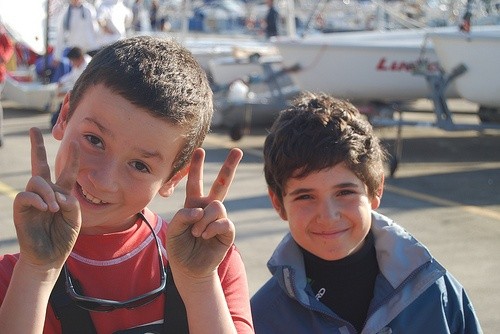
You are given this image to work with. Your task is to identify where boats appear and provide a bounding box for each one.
[0,1,500,144]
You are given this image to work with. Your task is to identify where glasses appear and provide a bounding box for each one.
[61,211,167,314]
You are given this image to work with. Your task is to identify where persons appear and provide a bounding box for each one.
[250,93,483,334]
[0,0,279,148]
[0,36,258,334]
